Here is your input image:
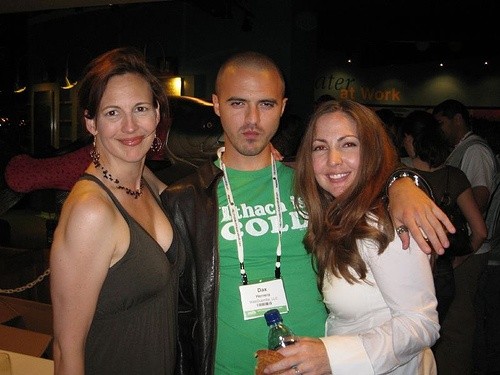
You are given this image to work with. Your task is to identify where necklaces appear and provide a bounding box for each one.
[90,149,146,200]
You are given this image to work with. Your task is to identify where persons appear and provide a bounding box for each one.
[264,98,441,375]
[375,98,500,375]
[160,51,456,375]
[50,48,284,375]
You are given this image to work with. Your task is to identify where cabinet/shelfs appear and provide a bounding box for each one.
[29,81,88,151]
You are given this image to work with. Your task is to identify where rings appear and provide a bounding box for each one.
[292,365,301,375]
[396,224,408,234]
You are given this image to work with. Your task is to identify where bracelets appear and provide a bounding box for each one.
[388,168,424,187]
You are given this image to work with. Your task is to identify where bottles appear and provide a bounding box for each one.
[264,309,300,351]
